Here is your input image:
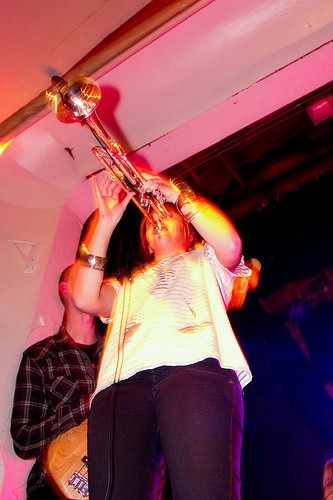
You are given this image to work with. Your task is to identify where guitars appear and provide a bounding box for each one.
[42,257,262,500]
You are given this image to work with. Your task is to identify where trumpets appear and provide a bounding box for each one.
[49,78,172,234]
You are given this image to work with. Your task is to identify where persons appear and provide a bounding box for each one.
[10,263,105,500]
[72,172,253,500]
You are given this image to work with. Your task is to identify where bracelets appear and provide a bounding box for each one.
[82,254,107,271]
[178,191,211,221]
